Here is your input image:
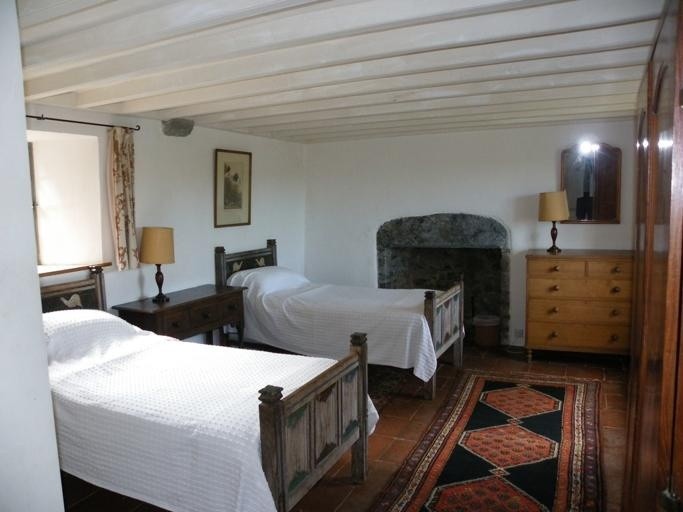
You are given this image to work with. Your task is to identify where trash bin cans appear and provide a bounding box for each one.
[473,313,500,348]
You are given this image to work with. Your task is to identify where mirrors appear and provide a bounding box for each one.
[559,139,621,225]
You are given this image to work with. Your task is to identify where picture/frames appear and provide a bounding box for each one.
[212,148,252,228]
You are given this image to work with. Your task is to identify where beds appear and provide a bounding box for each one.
[216,237,469,406]
[37,261,368,512]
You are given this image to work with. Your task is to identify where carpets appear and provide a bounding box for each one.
[370,368,607,511]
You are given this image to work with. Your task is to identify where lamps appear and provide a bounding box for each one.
[538,190,570,253]
[137,225,174,304]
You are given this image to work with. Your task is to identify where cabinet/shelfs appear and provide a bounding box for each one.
[523,247,635,365]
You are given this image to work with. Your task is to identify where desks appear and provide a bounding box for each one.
[110,284,248,347]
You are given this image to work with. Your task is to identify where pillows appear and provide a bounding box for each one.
[227,264,311,298]
